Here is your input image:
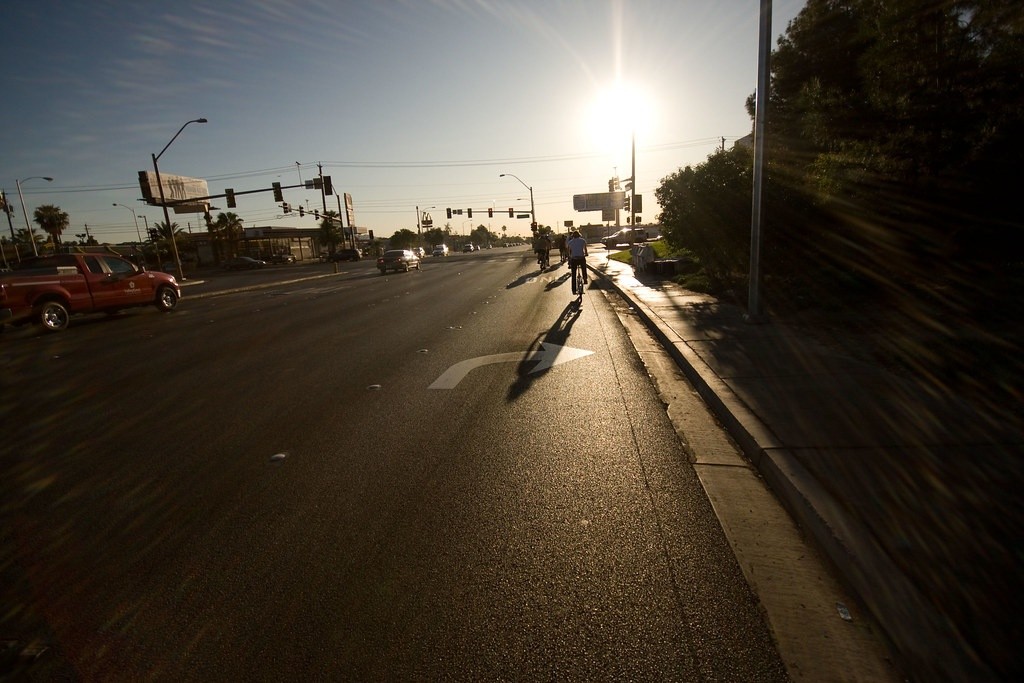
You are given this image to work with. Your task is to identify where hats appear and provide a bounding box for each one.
[571,231,582,238]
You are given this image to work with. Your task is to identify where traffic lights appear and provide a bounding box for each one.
[530,222,537,231]
[608,178,613,192]
[623,197,630,212]
[509,208,513,218]
[282,202,319,220]
[615,177,621,189]
[488,208,492,218]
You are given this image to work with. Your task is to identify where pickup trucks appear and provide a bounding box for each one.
[0,252,182,335]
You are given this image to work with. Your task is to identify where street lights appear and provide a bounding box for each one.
[149,117,208,281]
[15,176,55,256]
[111,203,151,247]
[416,206,435,234]
[499,173,535,237]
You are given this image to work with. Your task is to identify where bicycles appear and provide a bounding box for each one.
[569,254,588,303]
[534,249,551,273]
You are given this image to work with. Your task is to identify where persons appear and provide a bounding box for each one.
[534,235,551,269]
[567,230,588,295]
[559,232,573,262]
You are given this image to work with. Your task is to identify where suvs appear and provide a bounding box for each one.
[600,228,649,248]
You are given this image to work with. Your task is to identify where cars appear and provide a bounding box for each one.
[151,239,421,275]
[432,244,449,257]
[412,247,425,259]
[463,242,521,254]
